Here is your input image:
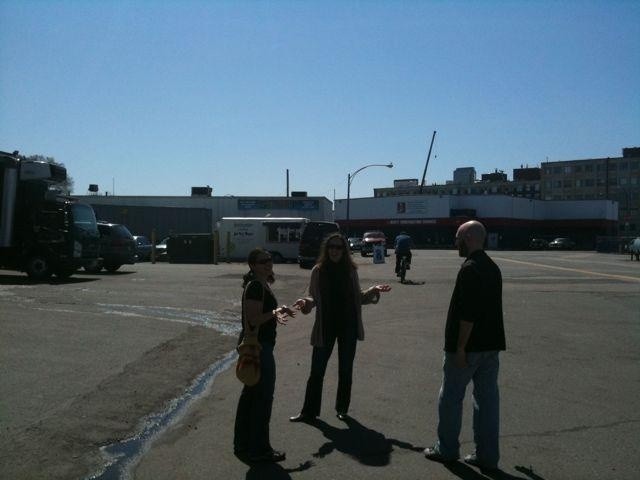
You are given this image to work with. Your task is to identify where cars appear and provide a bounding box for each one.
[527,234,572,250]
[348,237,362,251]
[133,236,174,263]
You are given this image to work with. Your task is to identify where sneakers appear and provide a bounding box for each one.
[464,453,477,463]
[425,447,458,462]
[271,449,286,461]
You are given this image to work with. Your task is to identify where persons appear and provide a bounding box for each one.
[234,249,298,468]
[422,217,508,470]
[290,232,392,425]
[393,231,415,273]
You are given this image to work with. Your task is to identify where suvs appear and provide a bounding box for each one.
[361,231,389,257]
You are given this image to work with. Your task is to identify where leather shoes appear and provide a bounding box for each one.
[337,412,344,419]
[289,412,317,423]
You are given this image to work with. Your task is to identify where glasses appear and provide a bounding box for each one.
[254,257,271,264]
[327,243,344,250]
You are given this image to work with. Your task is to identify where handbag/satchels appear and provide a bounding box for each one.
[236,333,262,384]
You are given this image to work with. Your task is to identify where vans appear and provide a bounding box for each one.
[75,220,136,273]
[297,221,342,268]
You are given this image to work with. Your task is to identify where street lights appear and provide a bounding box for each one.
[347,164,393,238]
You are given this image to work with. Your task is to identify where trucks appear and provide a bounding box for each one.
[217,216,311,264]
[0,150,101,284]
